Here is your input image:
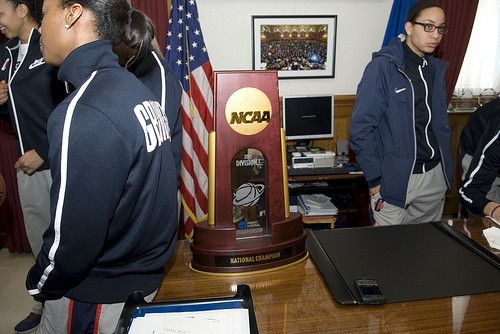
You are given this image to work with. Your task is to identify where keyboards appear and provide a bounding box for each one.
[289,167,349,176]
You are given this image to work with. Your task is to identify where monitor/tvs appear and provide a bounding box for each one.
[283,96,335,151]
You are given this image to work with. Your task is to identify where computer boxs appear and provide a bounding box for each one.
[286,147,336,169]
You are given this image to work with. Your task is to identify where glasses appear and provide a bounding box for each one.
[410,22,449,34]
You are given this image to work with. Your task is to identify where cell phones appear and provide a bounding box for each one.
[353,277,386,305]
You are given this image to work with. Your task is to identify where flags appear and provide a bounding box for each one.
[166,0,215,240]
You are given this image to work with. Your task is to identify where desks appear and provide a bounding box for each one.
[151,216,500,334]
[287,172,369,223]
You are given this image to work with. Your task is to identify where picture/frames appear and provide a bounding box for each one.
[251,14,338,80]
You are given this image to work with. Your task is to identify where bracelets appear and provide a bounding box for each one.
[490,204,500,218]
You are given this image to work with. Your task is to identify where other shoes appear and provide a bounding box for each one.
[15,312,42,333]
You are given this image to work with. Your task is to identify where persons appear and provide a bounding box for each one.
[458,97,500,225]
[0,0,182,334]
[349,3,456,227]
